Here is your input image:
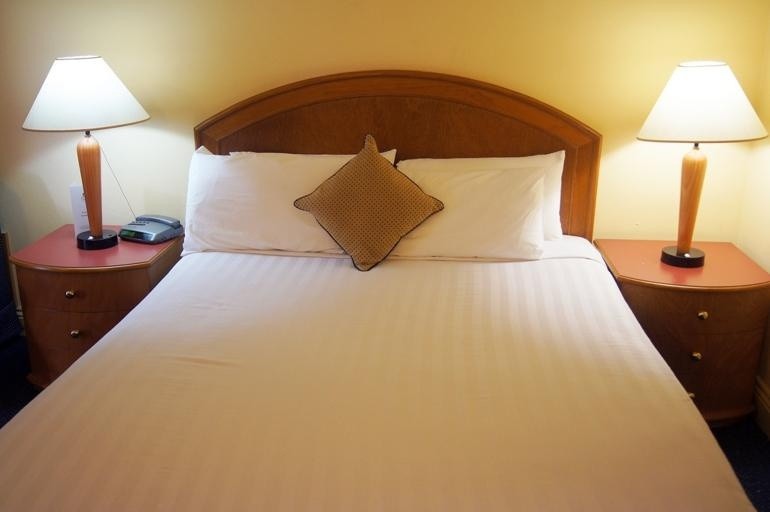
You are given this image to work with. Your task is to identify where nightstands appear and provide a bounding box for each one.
[592,235,770,432]
[7,222,185,391]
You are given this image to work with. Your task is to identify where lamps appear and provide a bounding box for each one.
[19,55,151,252]
[634,59,767,270]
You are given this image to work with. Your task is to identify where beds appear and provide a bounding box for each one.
[1,67,756,510]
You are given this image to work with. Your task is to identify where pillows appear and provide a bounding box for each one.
[390,166,545,265]
[396,149,566,241]
[179,144,399,258]
[292,135,445,272]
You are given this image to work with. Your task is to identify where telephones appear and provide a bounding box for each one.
[118,214,184,244]
